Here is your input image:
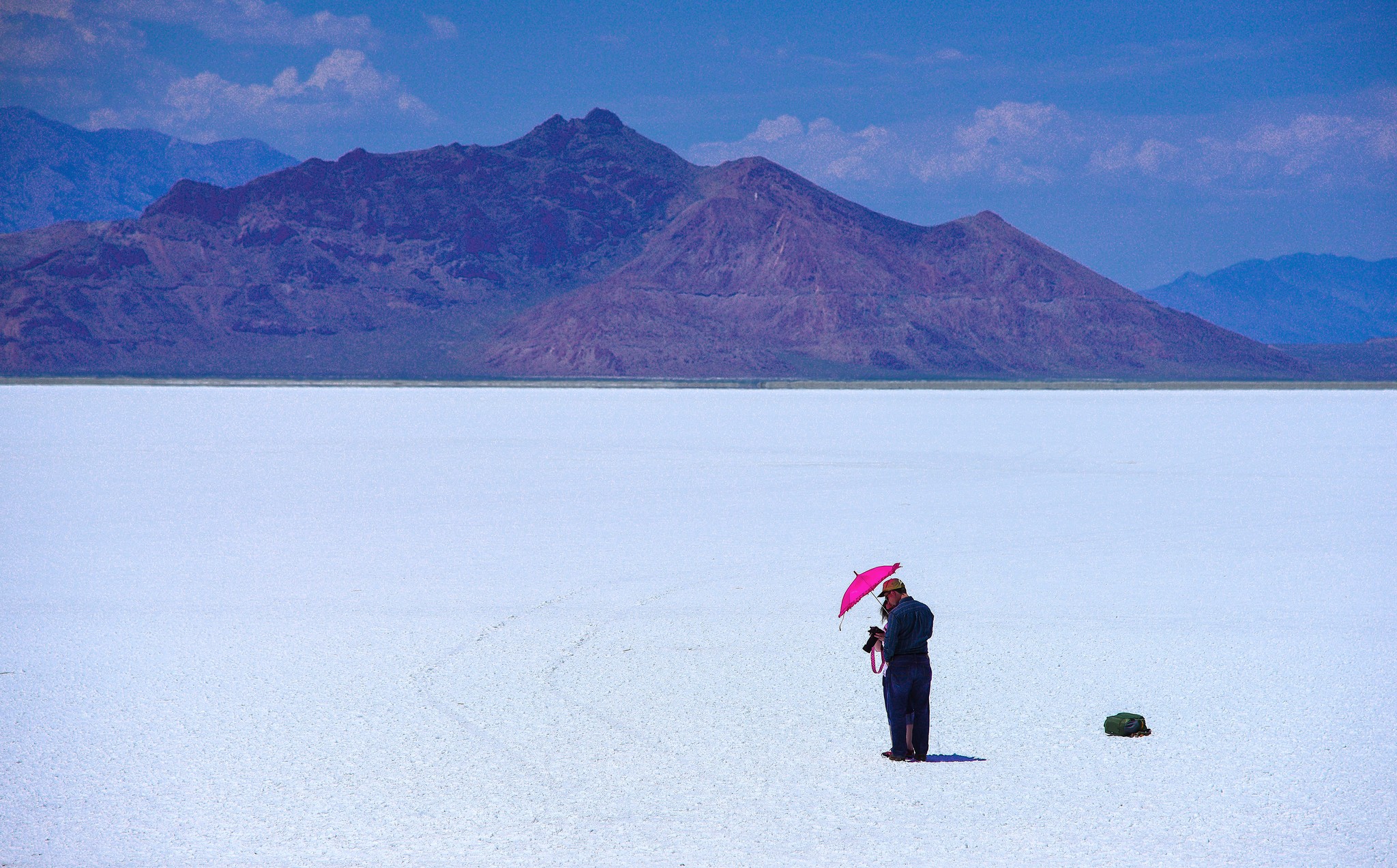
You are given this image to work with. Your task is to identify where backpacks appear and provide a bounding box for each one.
[1104,713,1152,737]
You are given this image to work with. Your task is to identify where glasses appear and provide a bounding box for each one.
[886,607,894,612]
[885,591,891,601]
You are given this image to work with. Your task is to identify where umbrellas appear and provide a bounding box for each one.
[839,563,903,631]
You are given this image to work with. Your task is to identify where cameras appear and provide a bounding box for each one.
[862,626,885,653]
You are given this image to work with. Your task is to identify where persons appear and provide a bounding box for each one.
[877,578,934,762]
[867,597,914,760]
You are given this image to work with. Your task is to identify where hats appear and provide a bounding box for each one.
[877,578,905,597]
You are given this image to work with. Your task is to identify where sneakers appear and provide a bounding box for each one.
[881,747,927,762]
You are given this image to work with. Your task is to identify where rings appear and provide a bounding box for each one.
[880,639,882,641]
